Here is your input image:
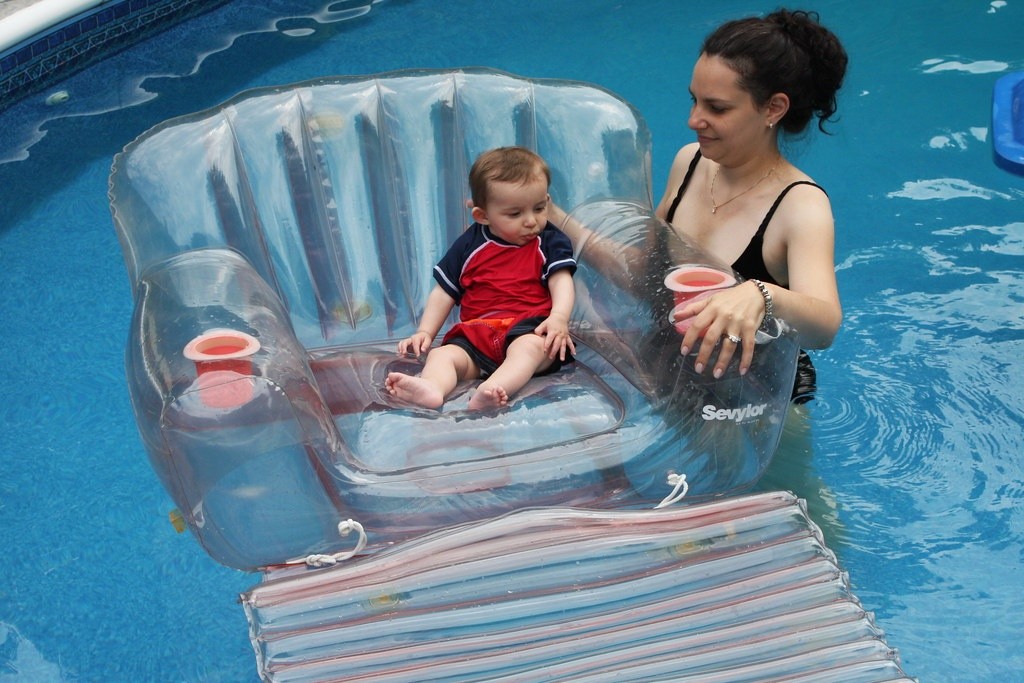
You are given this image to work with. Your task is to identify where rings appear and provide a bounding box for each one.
[727,334,742,343]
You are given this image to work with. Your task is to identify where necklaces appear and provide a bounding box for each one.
[709,165,775,214]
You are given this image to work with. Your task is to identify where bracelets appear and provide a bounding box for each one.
[749,279,774,329]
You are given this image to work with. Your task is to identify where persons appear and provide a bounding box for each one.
[466,8,847,379]
[385,147,576,410]
